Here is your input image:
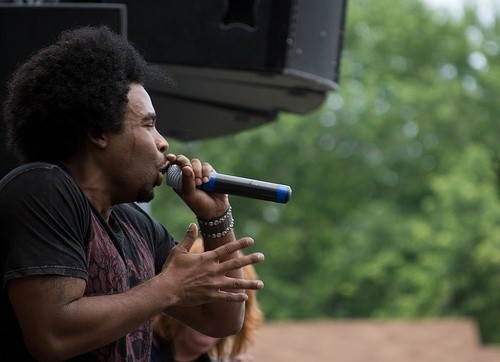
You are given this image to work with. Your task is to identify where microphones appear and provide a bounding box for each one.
[167,163,293,207]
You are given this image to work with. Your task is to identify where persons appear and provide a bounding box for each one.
[0,26,264,362]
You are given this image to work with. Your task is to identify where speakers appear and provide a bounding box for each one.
[0,0,351,146]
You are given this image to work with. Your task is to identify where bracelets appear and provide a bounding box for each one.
[195,205,234,239]
